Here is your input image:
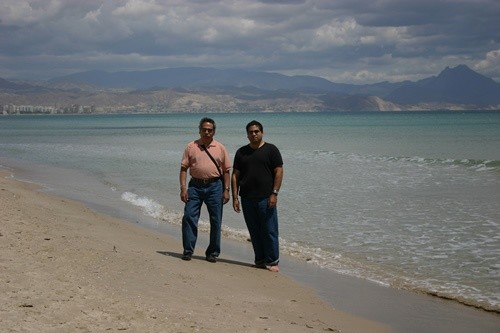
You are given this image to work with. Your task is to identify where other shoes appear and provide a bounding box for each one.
[182,252,192,260]
[206,254,216,263]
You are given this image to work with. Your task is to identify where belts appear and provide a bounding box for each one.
[192,176,222,184]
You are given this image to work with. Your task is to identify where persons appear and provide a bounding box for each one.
[230,120,283,272]
[179,118,232,263]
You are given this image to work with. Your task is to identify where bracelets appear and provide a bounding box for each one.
[225,186,229,192]
[272,190,278,196]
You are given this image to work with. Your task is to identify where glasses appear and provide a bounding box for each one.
[201,127,214,133]
[248,129,261,135]
